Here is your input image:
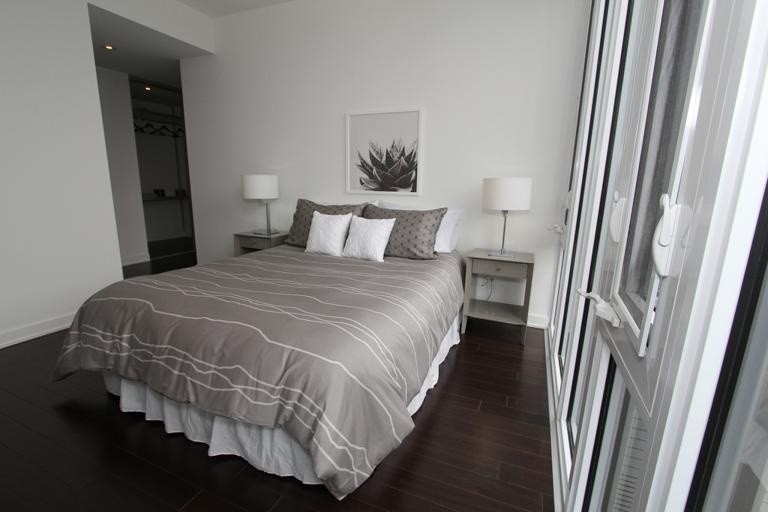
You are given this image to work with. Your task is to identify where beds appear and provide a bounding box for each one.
[50,250,468,501]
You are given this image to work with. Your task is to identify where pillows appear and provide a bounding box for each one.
[342,214,397,263]
[284,199,366,248]
[378,200,466,253]
[304,210,353,257]
[363,203,448,260]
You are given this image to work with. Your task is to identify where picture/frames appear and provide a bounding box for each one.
[346,105,425,195]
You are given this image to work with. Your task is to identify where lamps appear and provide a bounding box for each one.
[483,176,531,257]
[242,173,282,234]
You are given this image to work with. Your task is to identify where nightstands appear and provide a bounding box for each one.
[233,232,289,256]
[462,248,536,346]
